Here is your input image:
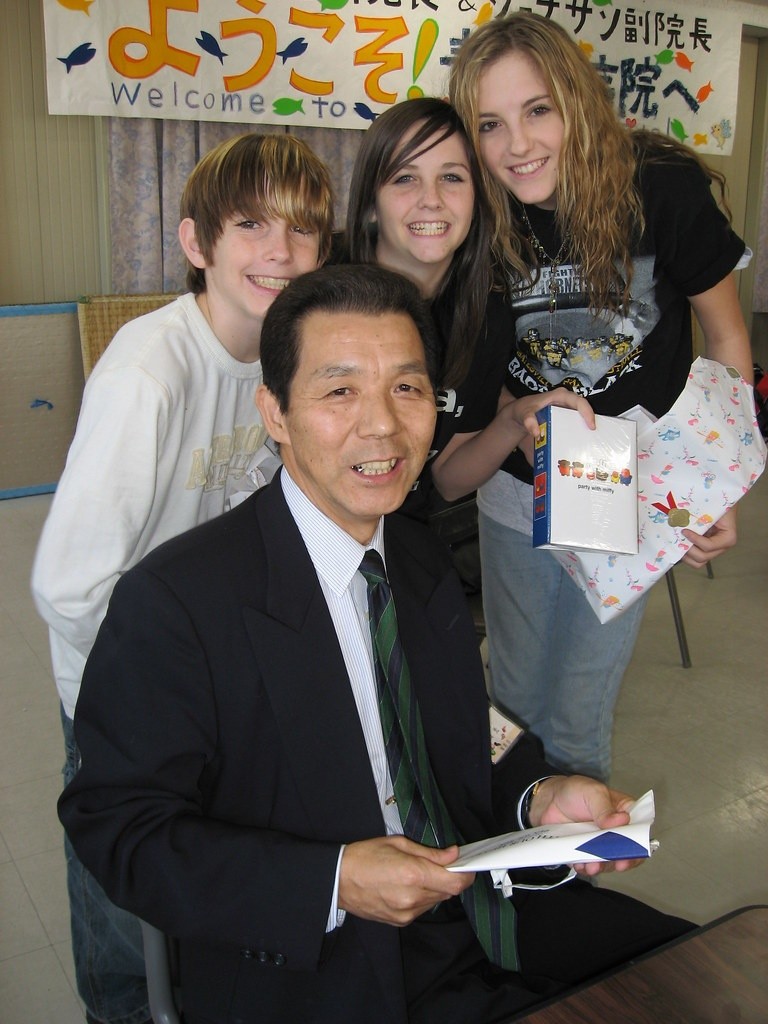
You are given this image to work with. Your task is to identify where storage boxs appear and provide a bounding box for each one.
[533,406,640,556]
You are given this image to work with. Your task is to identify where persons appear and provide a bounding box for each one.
[324,9,755,889]
[56,258,648,1024]
[30,132,335,1024]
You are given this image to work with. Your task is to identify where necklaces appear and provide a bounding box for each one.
[521,202,572,314]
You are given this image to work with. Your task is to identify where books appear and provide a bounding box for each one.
[532,404,638,557]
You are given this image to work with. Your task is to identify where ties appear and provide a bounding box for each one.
[345,549,523,974]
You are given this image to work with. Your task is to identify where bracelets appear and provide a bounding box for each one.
[521,777,553,829]
